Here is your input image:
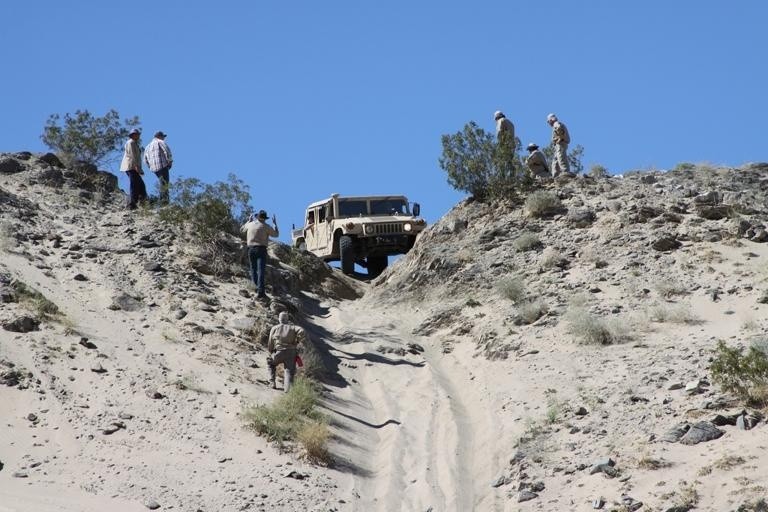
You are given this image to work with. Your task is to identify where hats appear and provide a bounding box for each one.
[153,130,167,137]
[128,128,142,137]
[279,312,289,322]
[526,143,539,151]
[254,209,269,221]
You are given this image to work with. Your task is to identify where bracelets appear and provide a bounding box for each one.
[248,220,251,222]
[274,224,277,227]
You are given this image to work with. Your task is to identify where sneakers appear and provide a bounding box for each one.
[269,381,277,388]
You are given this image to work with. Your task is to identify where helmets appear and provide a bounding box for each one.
[494,111,506,121]
[546,113,558,123]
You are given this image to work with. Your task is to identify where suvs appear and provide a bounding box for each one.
[291,193,427,273]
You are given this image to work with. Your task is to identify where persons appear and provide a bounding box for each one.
[545,113,571,179]
[119,128,154,210]
[523,143,550,180]
[240,209,280,299]
[493,110,516,179]
[143,131,174,204]
[266,310,306,394]
[304,214,314,230]
